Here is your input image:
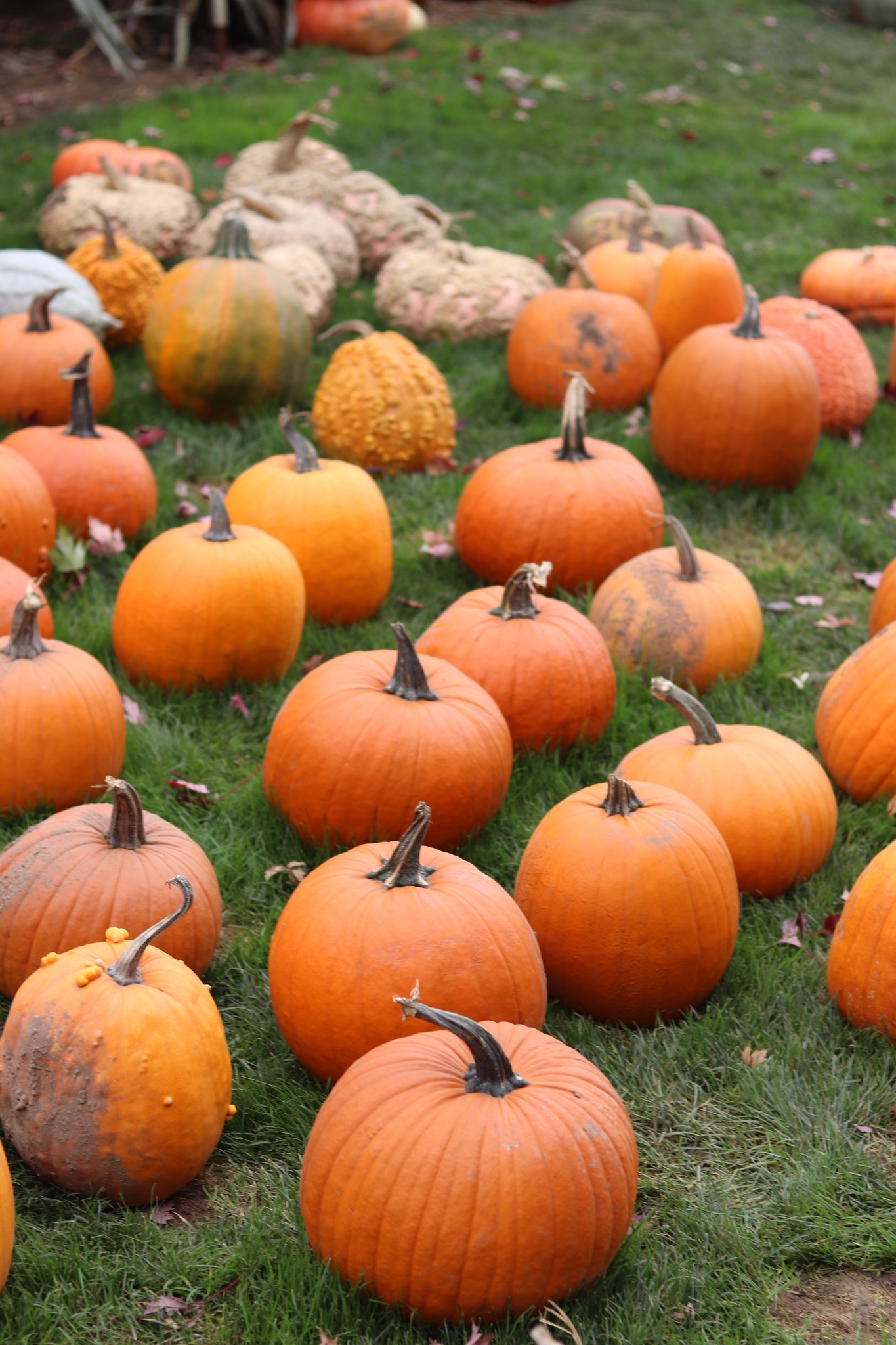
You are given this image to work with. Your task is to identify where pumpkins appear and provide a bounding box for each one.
[1,109,896,1322]
